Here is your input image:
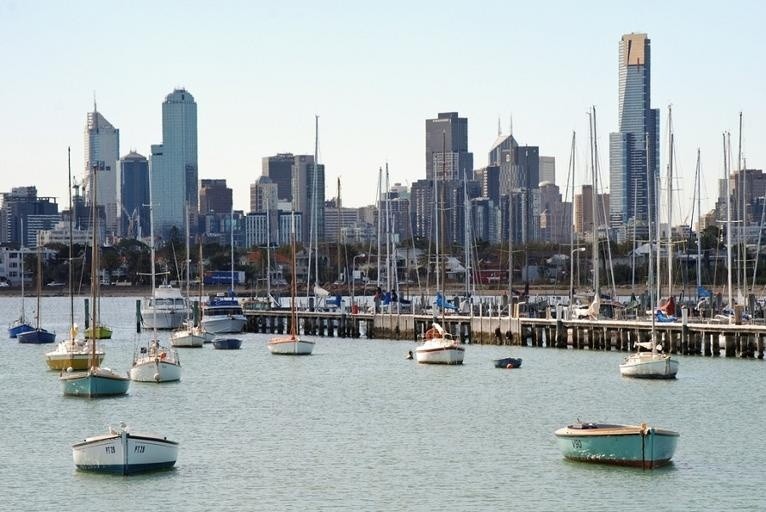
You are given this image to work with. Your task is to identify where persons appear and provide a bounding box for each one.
[630,289,651,311]
[392,288,397,300]
[373,286,382,314]
[406,351,413,359]
[383,292,390,314]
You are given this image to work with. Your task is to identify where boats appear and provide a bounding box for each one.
[68,425,181,474]
[555,417,679,472]
